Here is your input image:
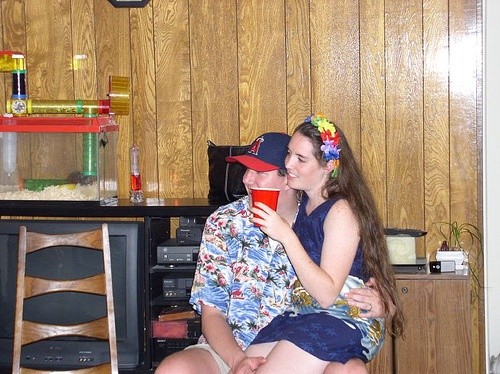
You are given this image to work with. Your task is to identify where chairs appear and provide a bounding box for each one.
[12,224,119,374]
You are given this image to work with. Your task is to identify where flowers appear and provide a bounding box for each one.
[304,113,342,178]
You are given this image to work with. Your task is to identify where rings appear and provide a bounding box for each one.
[368,302,373,312]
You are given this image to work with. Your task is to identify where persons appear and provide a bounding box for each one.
[228,113,406,374]
[154,132,396,374]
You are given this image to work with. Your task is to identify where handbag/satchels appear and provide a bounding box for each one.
[206,139,251,202]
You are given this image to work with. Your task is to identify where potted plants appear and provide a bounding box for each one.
[432,221,485,304]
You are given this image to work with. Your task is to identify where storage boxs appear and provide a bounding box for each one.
[152,320,188,339]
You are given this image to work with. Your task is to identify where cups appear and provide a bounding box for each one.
[249,187,281,228]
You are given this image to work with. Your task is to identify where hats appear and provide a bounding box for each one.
[225,132,292,172]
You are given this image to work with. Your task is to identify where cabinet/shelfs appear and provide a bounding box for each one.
[0,198,217,374]
[365,273,474,373]
